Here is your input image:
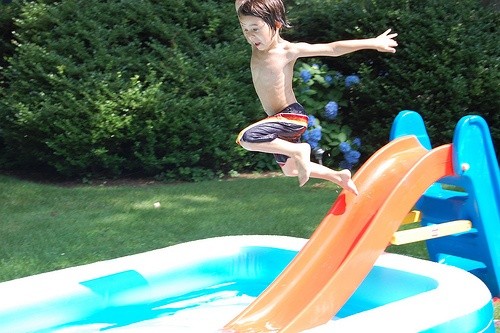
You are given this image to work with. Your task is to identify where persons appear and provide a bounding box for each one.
[235,0,398,196]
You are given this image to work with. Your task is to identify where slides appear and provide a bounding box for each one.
[220,133,455,333]
